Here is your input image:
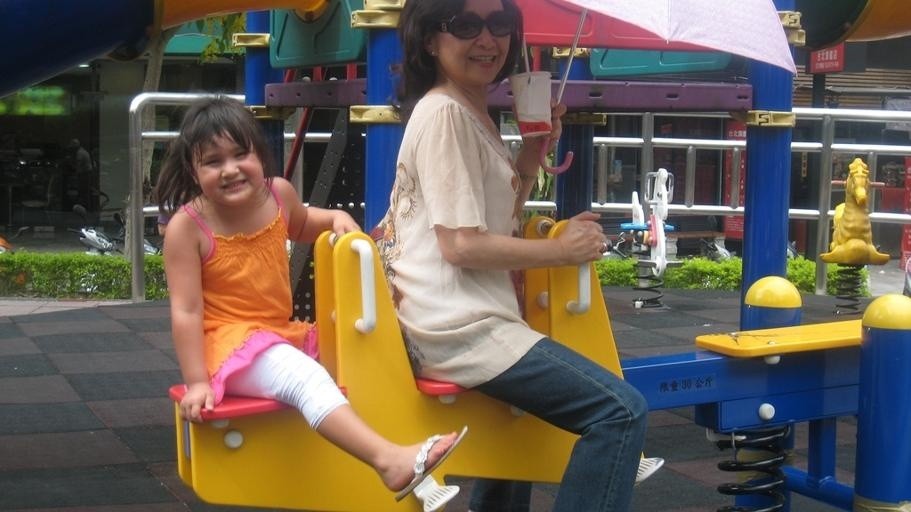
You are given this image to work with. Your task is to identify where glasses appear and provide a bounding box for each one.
[437,11,516,39]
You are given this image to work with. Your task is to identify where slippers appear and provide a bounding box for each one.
[395,423,469,503]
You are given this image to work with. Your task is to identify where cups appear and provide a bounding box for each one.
[507,69,553,140]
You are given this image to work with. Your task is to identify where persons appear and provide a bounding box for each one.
[162,94,470,501]
[378,0,650,512]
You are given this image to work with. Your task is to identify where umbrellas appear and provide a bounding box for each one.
[509,0,798,174]
